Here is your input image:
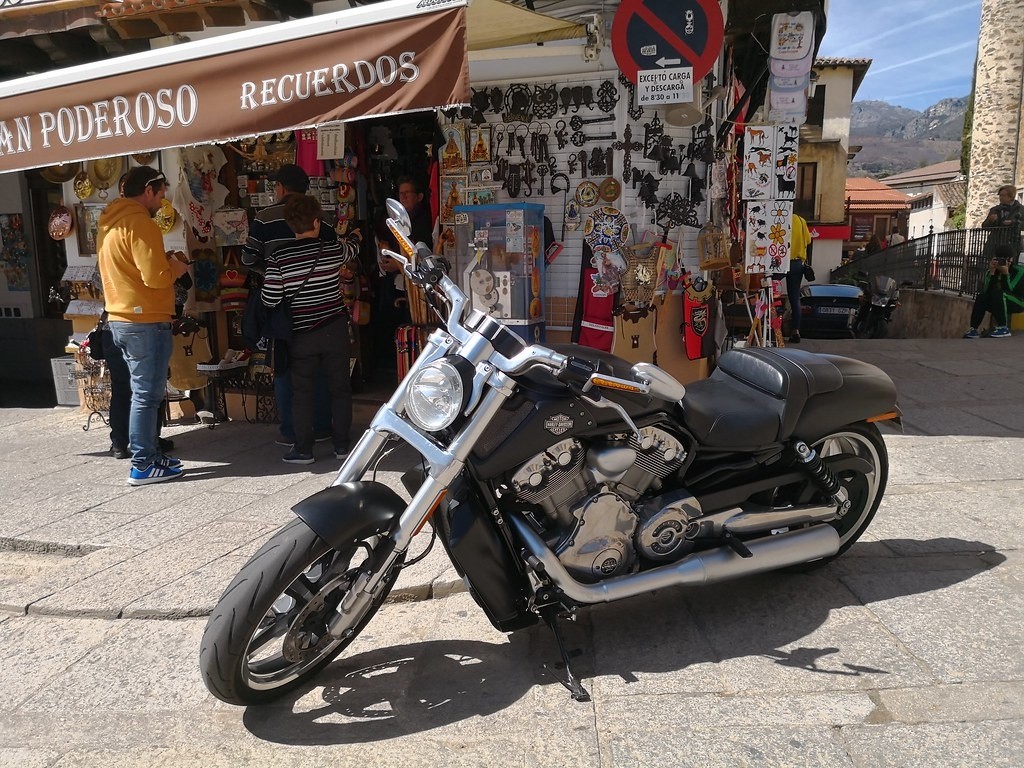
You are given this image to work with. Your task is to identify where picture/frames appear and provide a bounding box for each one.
[73,202,107,257]
[440,121,495,224]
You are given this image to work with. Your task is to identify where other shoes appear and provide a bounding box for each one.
[275,438,295,446]
[109,444,128,458]
[788,334,800,344]
[312,430,332,441]
[159,438,174,452]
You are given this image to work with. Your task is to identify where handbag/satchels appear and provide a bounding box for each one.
[803,265,816,283]
[221,287,249,311]
[88,329,106,360]
[218,250,248,286]
[256,296,294,340]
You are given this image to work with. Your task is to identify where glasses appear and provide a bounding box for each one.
[145,172,166,187]
[399,190,417,197]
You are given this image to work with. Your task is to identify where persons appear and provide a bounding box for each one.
[851,243,868,260]
[760,213,812,344]
[982,185,1024,260]
[865,234,882,256]
[260,195,363,464]
[379,176,433,324]
[95,166,190,484]
[963,245,1024,338]
[882,227,904,249]
[240,164,337,446]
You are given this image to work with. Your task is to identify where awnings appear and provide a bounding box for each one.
[0,0,587,174]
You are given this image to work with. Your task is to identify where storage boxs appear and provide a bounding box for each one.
[51,356,79,406]
[197,359,249,370]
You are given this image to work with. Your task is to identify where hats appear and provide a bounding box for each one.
[267,164,308,193]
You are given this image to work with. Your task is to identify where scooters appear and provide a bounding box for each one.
[846,270,914,339]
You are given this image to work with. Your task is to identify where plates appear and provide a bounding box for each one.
[38,153,174,240]
[564,176,630,254]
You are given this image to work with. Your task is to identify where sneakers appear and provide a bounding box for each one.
[129,463,183,485]
[333,448,350,459]
[152,455,181,468]
[964,327,979,338]
[990,326,1011,337]
[282,447,315,464]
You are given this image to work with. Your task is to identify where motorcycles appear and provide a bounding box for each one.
[198,195,906,707]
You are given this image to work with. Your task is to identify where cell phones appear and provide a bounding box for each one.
[184,260,196,266]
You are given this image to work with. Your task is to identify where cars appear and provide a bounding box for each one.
[798,283,865,339]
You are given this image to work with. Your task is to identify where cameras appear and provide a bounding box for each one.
[994,257,1007,266]
